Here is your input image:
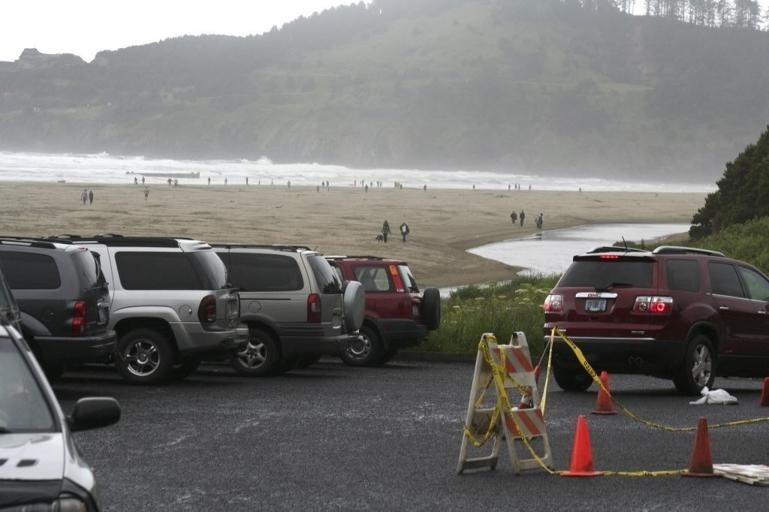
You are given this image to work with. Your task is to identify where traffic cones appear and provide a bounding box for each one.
[756,375,769,407]
[673,413,721,480]
[509,367,548,413]
[556,413,607,480]
[591,368,617,417]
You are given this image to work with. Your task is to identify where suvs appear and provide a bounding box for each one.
[538,233,769,399]
[0,319,131,512]
[0,233,118,385]
[50,233,248,386]
[326,254,443,368]
[195,241,366,378]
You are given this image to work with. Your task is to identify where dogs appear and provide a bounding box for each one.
[375,234,383,243]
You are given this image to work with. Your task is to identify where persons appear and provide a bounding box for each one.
[376,233,383,241]
[520,209,527,227]
[511,210,517,223]
[144,186,150,200]
[399,221,409,242]
[80,188,88,204]
[89,189,94,205]
[381,220,390,242]
[538,212,545,229]
[535,215,540,227]
